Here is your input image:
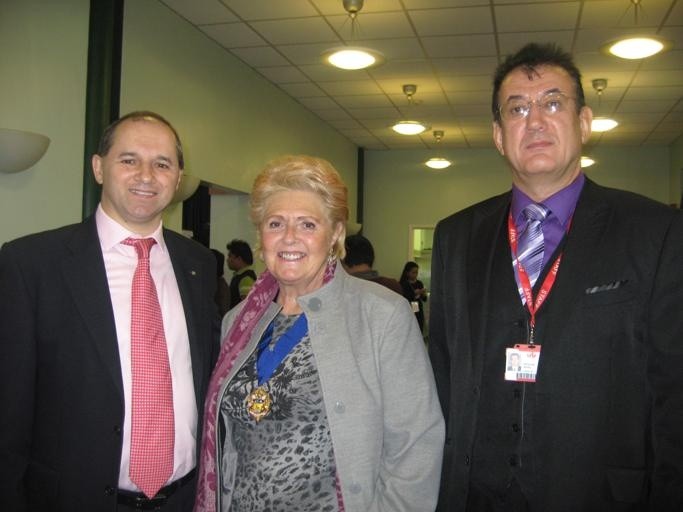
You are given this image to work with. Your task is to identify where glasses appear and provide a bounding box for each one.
[494,90,585,126]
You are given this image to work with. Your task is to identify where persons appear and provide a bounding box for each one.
[1,111,226,510]
[209,237,429,334]
[426,42,683,512]
[193,154,446,511]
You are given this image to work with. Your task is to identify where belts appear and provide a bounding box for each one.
[122,493,174,511]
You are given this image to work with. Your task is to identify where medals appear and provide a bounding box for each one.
[244,313,308,422]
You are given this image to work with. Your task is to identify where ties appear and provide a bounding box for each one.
[121,237,175,502]
[511,202,551,306]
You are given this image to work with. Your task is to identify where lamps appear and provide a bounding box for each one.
[597,0,671,64]
[388,81,433,138]
[588,76,619,135]
[422,128,453,172]
[316,0,390,76]
